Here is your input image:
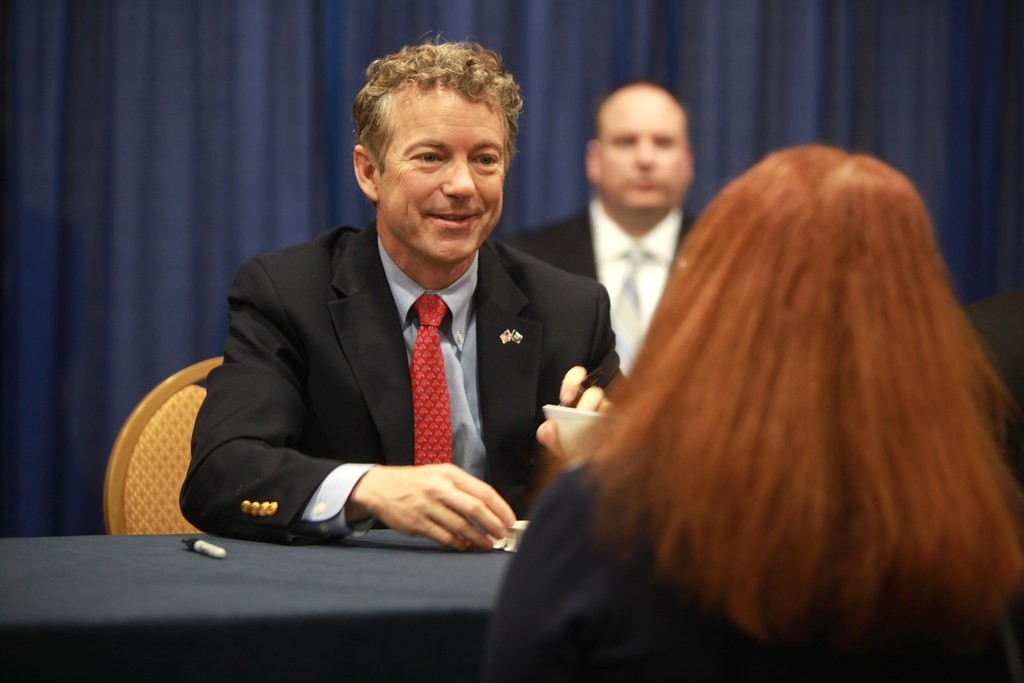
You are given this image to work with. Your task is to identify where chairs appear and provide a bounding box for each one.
[103,356,227,535]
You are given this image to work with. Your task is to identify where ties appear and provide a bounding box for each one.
[409,295,454,464]
[612,253,646,374]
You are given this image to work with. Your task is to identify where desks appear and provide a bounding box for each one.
[0,530,513,683]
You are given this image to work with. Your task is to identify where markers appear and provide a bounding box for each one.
[181,537,228,559]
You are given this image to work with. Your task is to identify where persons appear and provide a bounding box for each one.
[488,142,1023,683]
[506,81,700,378]
[177,34,625,552]
